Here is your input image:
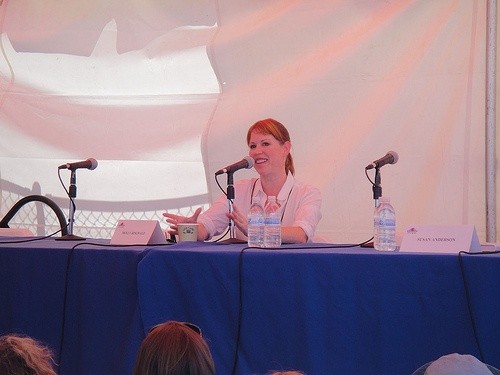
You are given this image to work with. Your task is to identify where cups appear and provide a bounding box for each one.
[177,223,198,244]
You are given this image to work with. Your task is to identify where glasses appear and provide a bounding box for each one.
[148,322,200,335]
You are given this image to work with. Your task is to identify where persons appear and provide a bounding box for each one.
[411,353,500,375]
[134,320,216,375]
[163,118,323,243]
[0,333,59,375]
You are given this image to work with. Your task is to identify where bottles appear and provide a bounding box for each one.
[263,196,281,248]
[373,196,396,253]
[247,198,265,248]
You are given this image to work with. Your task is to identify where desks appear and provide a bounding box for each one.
[0,236,500,375]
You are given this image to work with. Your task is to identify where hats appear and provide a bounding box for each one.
[412,353,500,375]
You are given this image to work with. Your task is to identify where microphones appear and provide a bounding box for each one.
[365,150,399,170]
[58,158,98,170]
[214,155,255,175]
